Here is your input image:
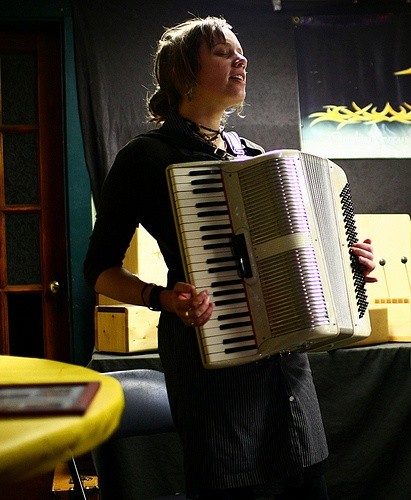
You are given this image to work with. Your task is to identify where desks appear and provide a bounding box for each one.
[92,342,411,500]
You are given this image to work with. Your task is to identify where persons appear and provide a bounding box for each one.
[86,15,379,500]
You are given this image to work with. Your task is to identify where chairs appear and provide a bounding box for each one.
[92,368,186,500]
[0,355,126,500]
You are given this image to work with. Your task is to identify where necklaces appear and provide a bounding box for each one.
[198,125,220,143]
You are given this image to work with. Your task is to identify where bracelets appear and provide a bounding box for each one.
[141,283,165,310]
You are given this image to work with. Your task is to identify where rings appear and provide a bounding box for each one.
[192,322,195,326]
[185,310,189,318]
